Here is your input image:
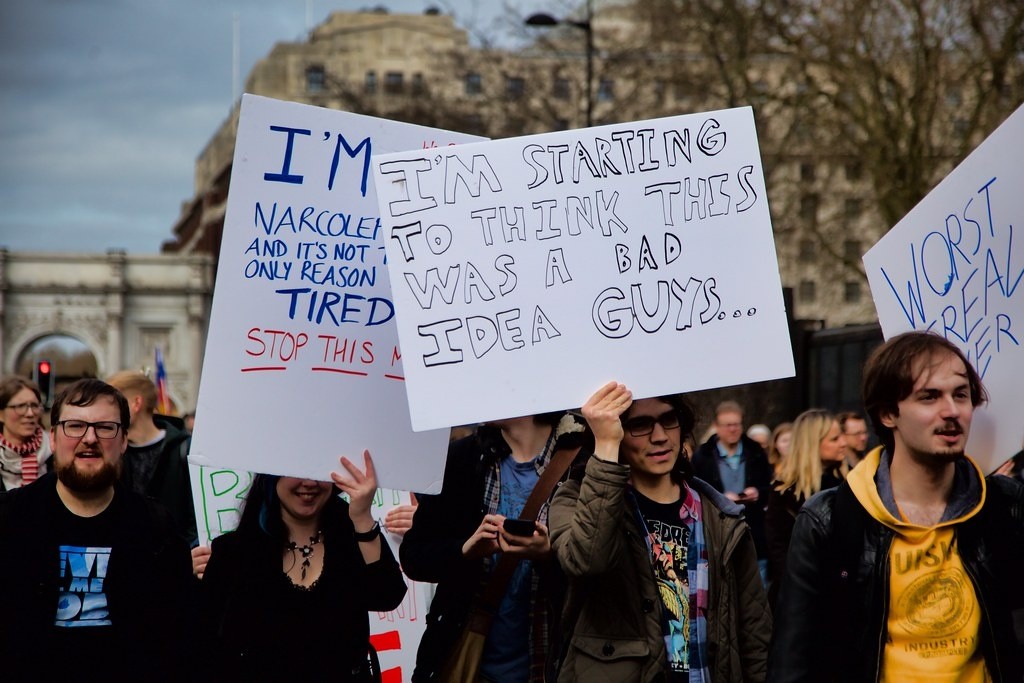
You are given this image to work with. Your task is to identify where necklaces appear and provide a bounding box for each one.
[286,529,321,581]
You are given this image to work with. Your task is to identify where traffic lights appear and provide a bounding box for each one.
[33,361,55,410]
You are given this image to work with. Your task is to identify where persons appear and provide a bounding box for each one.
[400,383,1024,683]
[106,368,189,495]
[766,331,1024,683]
[181,411,417,682]
[0,381,196,683]
[0,377,55,492]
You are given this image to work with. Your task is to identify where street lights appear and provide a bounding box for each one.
[527,13,594,129]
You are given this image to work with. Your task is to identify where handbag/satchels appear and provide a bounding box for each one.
[411,611,490,683]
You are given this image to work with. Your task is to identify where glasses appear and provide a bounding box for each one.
[6,405,41,415]
[56,421,121,438]
[623,412,682,438]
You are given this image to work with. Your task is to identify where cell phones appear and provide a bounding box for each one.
[738,493,746,497]
[501,519,536,546]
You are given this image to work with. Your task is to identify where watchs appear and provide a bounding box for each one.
[355,521,380,541]
